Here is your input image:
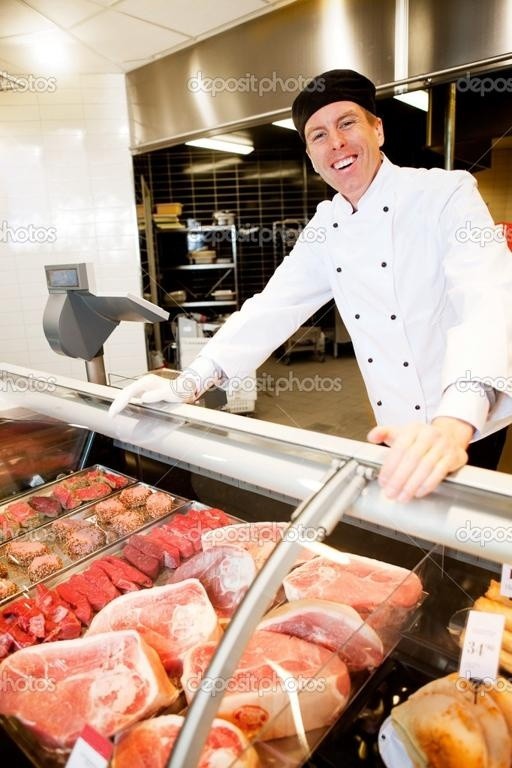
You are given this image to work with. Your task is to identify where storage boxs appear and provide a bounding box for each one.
[136,204,155,218]
[157,203,183,214]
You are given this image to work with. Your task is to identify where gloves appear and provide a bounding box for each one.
[108,376,196,421]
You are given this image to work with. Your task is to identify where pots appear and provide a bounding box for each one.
[213,209,236,228]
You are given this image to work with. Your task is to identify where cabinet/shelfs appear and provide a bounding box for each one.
[138,225,240,350]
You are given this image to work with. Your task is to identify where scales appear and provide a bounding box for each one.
[43,262,227,409]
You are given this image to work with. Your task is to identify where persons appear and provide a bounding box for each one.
[106,69,511,502]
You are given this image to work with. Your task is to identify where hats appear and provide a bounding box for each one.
[291,70,377,147]
